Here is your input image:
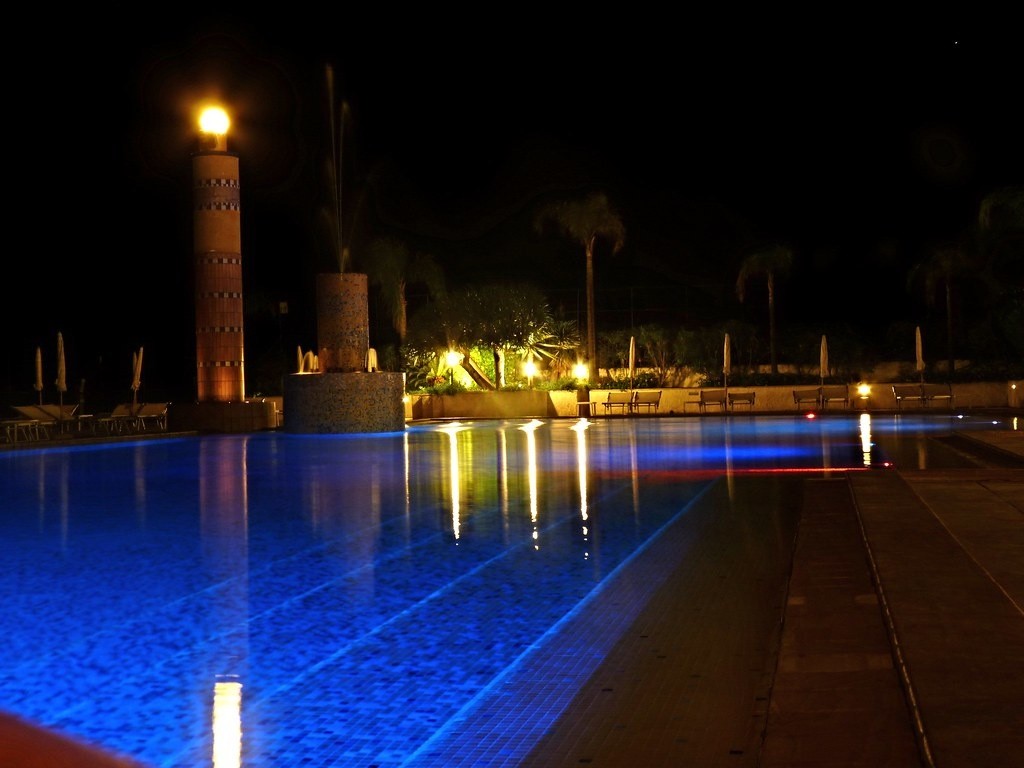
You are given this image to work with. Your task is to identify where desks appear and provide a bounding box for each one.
[1,420,40,443]
[85,417,117,435]
[576,402,597,416]
[684,401,706,412]
[850,394,871,409]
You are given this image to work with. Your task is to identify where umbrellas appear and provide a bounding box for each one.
[628,335,635,388]
[131,346,144,402]
[723,332,730,387]
[916,326,926,383]
[33,347,44,405]
[55,331,68,406]
[819,335,830,386]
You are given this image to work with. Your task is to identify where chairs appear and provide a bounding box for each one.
[793,385,849,411]
[602,390,662,415]
[700,390,755,412]
[11,403,97,437]
[892,383,953,410]
[98,400,172,435]
